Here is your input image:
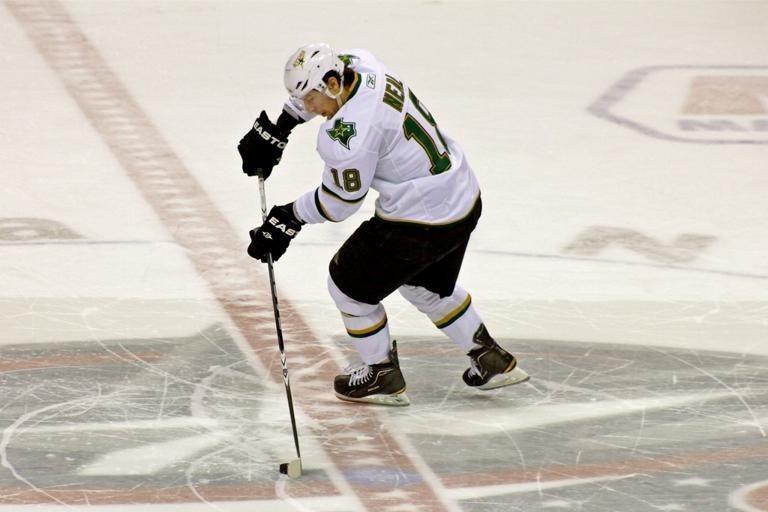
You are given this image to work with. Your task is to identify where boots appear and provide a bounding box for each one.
[460,324,518,388]
[333,339,407,400]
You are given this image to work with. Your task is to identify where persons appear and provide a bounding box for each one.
[234,41,519,400]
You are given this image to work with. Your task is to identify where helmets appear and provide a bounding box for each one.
[282,40,348,101]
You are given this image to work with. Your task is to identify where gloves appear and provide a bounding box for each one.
[236,109,289,180]
[249,205,303,264]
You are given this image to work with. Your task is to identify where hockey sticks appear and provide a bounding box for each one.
[256,167,301,479]
